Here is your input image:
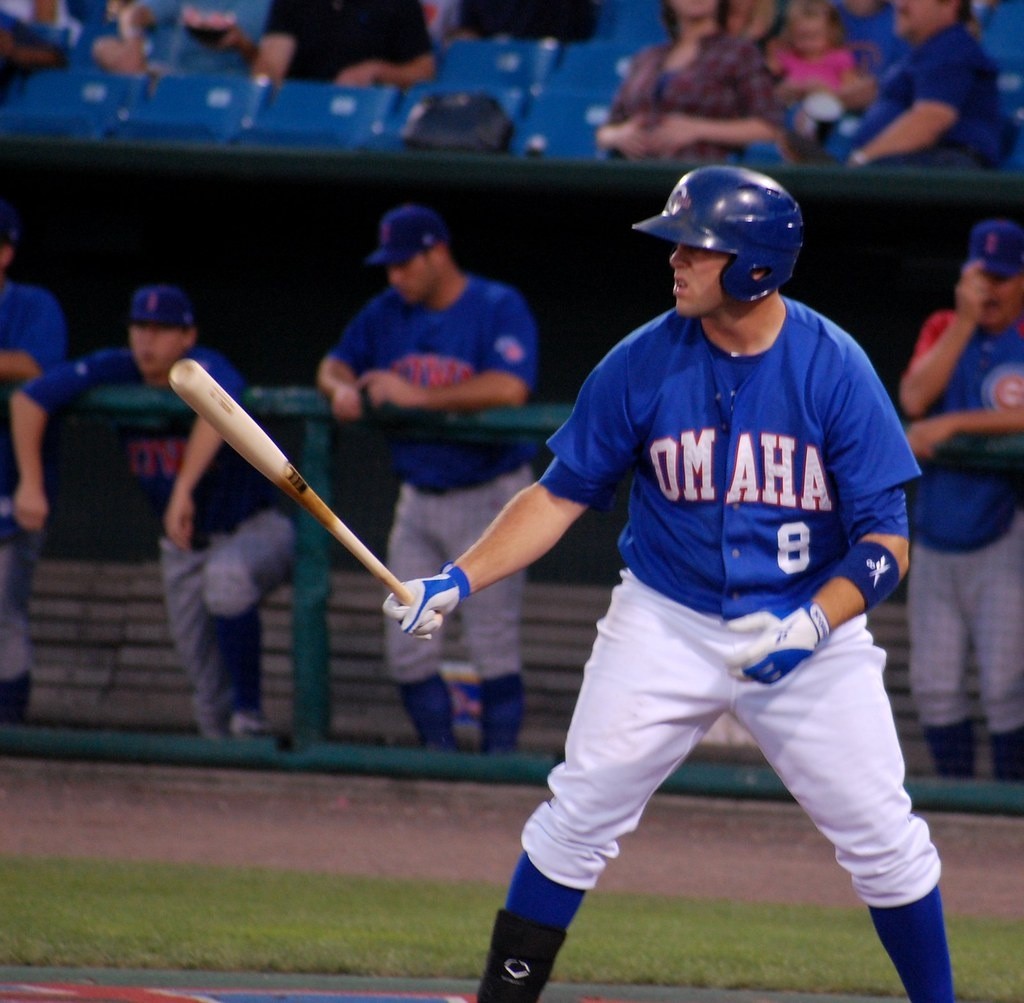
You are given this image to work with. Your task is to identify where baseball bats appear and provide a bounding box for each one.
[167,357,443,635]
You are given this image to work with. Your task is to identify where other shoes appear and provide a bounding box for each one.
[228,707,272,739]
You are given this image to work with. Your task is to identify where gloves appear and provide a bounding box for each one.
[383,563,471,641]
[721,594,832,685]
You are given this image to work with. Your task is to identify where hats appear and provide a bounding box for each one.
[961,217,1024,278]
[131,283,192,327]
[0,206,20,245]
[367,204,448,265]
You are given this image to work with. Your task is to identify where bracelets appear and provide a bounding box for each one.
[846,151,868,166]
[833,541,900,614]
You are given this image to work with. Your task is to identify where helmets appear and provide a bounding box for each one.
[631,163,804,303]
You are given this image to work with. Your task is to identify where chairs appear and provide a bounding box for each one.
[0,0,1024,184]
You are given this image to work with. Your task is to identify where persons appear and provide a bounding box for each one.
[0,245,76,720]
[9,288,298,737]
[0,0,1024,174]
[901,222,1022,788]
[317,206,543,755]
[377,161,956,1003]
